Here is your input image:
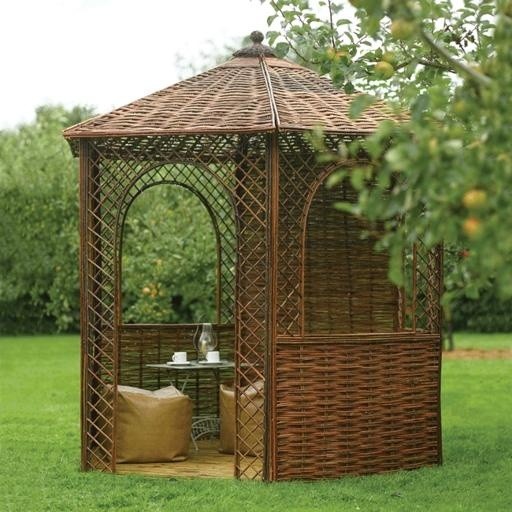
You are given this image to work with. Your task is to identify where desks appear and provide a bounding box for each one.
[145,359,261,452]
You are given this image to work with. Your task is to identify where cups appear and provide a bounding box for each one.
[171,351,187,361]
[205,351,220,362]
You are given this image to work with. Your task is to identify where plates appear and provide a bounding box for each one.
[166,361,192,366]
[198,360,224,366]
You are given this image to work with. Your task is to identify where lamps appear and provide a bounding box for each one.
[192,311,219,365]
